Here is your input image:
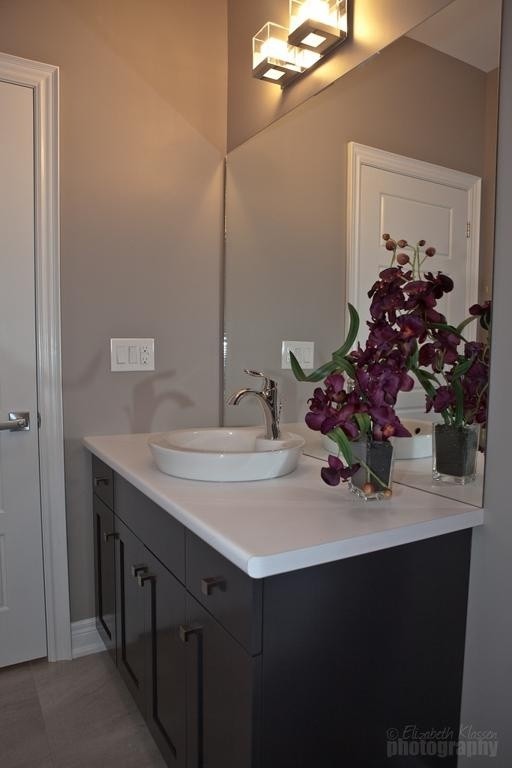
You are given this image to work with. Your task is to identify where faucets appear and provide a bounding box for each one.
[226,366,283,440]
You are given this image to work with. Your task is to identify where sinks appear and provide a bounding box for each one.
[323,418,438,460]
[148,427,306,482]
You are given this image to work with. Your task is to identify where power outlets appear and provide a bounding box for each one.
[282,340,294,369]
[138,338,154,372]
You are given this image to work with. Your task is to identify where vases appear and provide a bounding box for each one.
[431,421,480,485]
[348,441,394,503]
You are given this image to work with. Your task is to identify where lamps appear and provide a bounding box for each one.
[251,0,350,90]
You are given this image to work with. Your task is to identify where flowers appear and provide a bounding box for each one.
[290,234,428,492]
[412,239,492,429]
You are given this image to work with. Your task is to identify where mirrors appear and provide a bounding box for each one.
[221,1,504,509]
